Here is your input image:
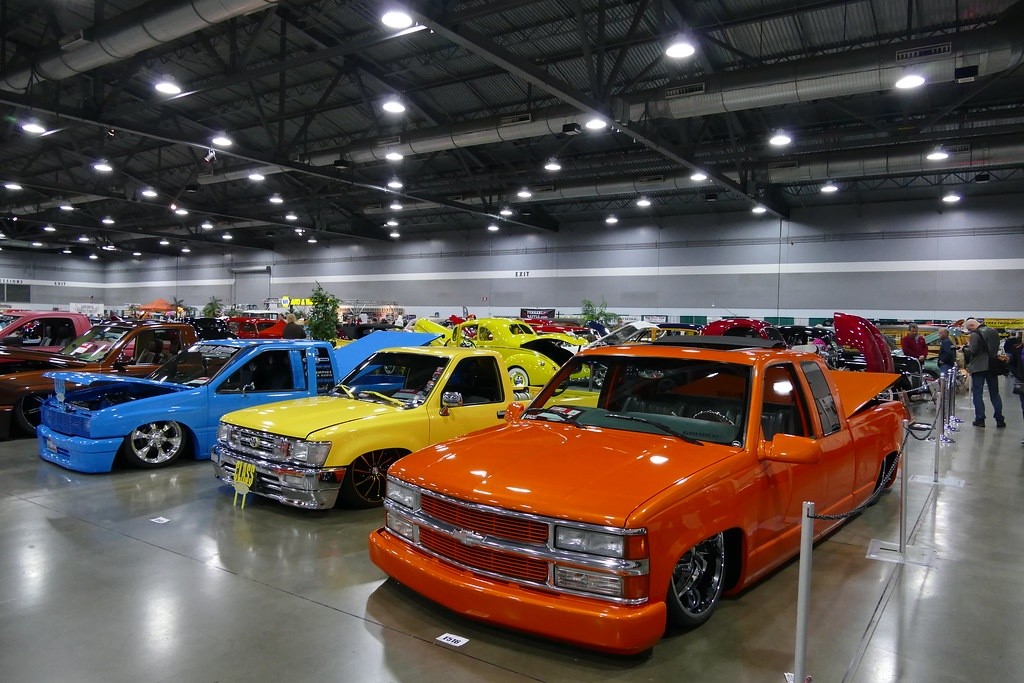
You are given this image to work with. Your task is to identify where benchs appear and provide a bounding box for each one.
[620,382,799,442]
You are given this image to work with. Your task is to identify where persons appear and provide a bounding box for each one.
[1008,329,1024,444]
[613,318,624,331]
[178,306,186,318]
[281,314,307,339]
[1003,331,1021,377]
[812,336,830,349]
[958,319,1006,427]
[901,323,928,386]
[937,328,956,378]
[276,314,286,323]
[343,313,403,326]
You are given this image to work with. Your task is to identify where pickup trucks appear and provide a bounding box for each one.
[211,346,600,509]
[370,335,908,655]
[37,331,445,473]
[411,312,964,392]
[0,313,289,436]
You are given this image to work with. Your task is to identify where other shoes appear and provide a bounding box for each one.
[996,420,1006,428]
[972,420,986,427]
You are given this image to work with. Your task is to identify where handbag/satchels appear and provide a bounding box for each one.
[989,356,1011,375]
[1012,383,1024,394]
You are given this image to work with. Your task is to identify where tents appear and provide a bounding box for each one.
[138,299,176,320]
